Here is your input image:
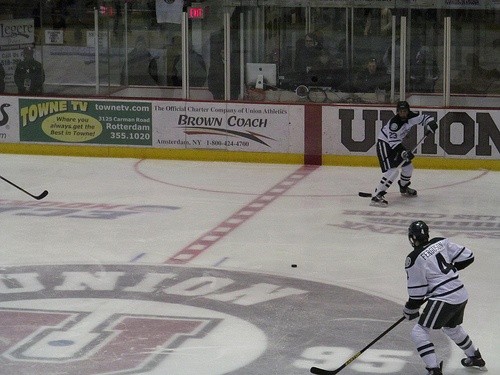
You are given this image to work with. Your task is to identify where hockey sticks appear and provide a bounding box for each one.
[359,130,430,197]
[310,298,428,375]
[0,175,48,200]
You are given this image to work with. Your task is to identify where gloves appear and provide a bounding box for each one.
[424,115,438,134]
[401,150,416,163]
[403,297,423,320]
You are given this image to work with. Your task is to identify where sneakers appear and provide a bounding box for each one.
[460,349,486,368]
[397,180,419,196]
[369,190,389,208]
[425,361,444,375]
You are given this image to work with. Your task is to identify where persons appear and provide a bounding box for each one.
[1,0,500,97]
[368,100,439,207]
[402,220,486,375]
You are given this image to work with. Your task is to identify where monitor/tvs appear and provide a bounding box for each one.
[245,63,277,89]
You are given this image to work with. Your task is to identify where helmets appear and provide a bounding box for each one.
[407,220,430,243]
[396,100,409,112]
